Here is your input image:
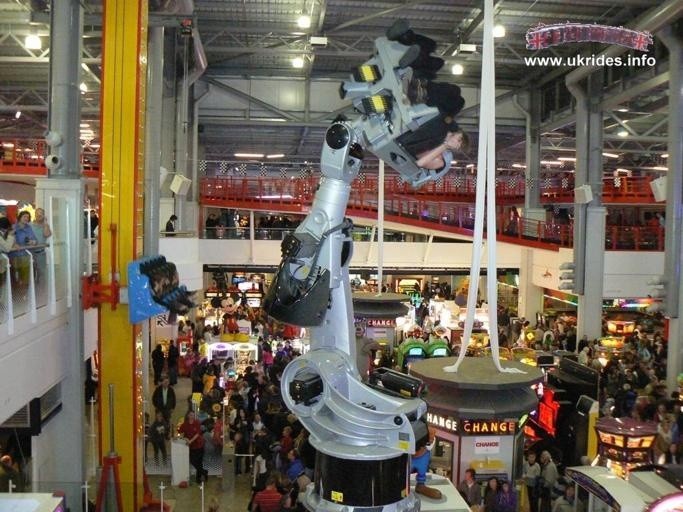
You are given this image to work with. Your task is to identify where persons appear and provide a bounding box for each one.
[89,209,98,245]
[415,127,467,180]
[0,217,17,311]
[142,273,683,512]
[8,211,37,301]
[412,198,665,247]
[165,214,177,237]
[28,208,51,293]
[206,212,293,240]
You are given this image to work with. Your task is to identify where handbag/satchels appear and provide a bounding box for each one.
[234,432,243,441]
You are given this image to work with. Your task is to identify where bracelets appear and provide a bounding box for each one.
[444,139,447,149]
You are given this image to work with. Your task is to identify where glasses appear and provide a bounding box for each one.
[1,226,12,228]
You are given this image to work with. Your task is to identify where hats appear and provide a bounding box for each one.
[0,218,12,225]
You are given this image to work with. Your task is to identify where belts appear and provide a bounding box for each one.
[33,249,44,254]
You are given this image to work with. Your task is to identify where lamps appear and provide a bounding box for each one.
[80,82,88,96]
[614,125,629,138]
[80,123,100,149]
[291,53,304,69]
[296,0,311,29]
[24,26,41,50]
[255,194,294,199]
[14,110,22,121]
[618,102,629,113]
[2,143,44,161]
[234,152,285,159]
[493,7,506,37]
[451,152,669,174]
[449,47,465,76]
